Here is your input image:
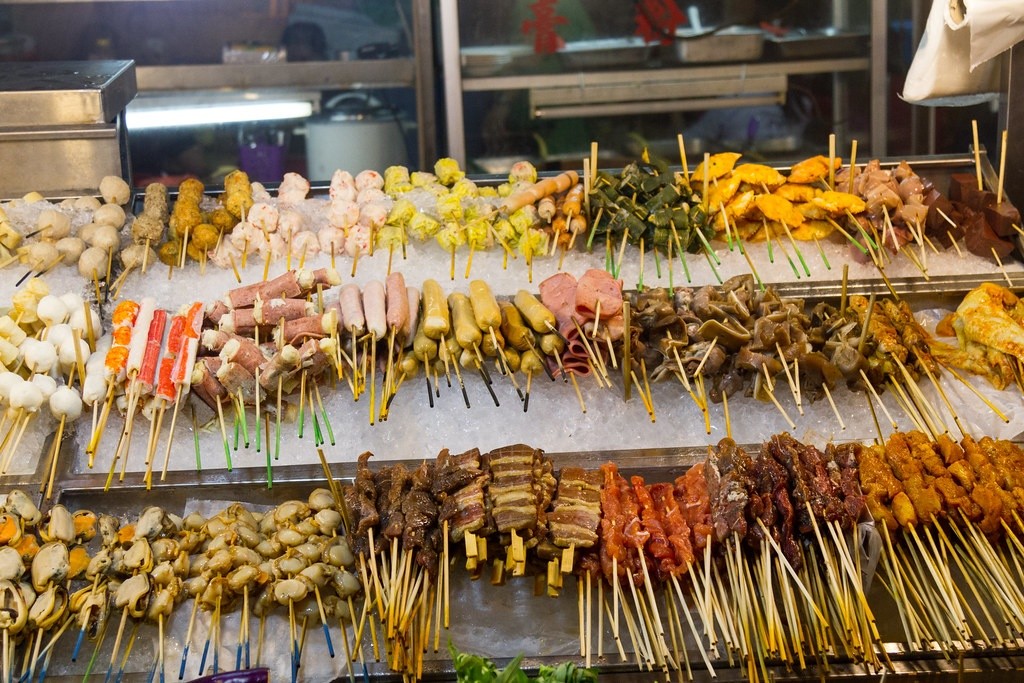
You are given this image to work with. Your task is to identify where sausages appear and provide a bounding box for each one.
[501,168,586,246]
[339,272,562,381]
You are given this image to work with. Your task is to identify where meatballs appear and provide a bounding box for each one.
[120,182,168,269]
[29,176,128,279]
[159,156,551,266]
[4,294,107,419]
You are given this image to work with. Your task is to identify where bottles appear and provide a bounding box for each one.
[237,118,284,177]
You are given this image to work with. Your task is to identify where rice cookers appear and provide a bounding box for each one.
[290,92,418,190]
